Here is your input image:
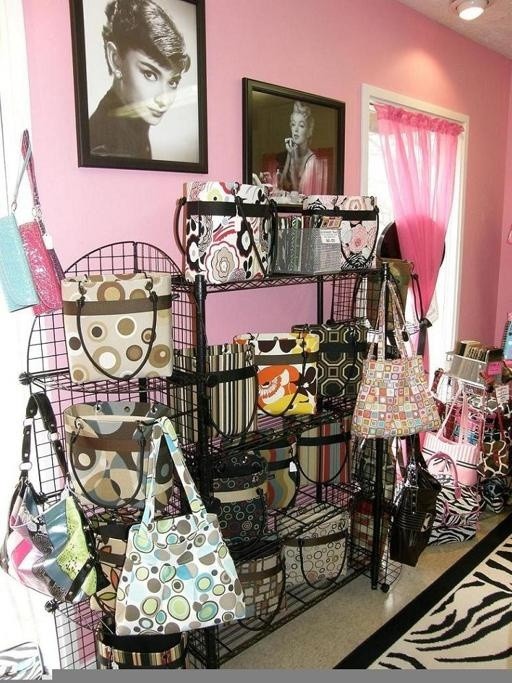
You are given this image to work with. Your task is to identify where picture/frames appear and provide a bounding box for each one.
[242,75,347,230]
[69,1,210,176]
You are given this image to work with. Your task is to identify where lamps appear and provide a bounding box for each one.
[448,0,495,21]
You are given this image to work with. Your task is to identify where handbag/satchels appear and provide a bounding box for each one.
[176,318,369,437]
[60,273,174,385]
[0,395,351,663]
[347,280,441,569]
[183,180,380,284]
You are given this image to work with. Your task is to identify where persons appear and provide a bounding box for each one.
[276,101,318,194]
[87,0,190,163]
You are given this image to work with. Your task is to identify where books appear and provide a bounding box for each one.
[273,214,346,275]
[451,339,503,387]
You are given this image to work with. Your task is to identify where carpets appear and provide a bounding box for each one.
[332,513,510,671]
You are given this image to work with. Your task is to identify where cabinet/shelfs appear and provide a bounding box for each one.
[17,239,391,671]
